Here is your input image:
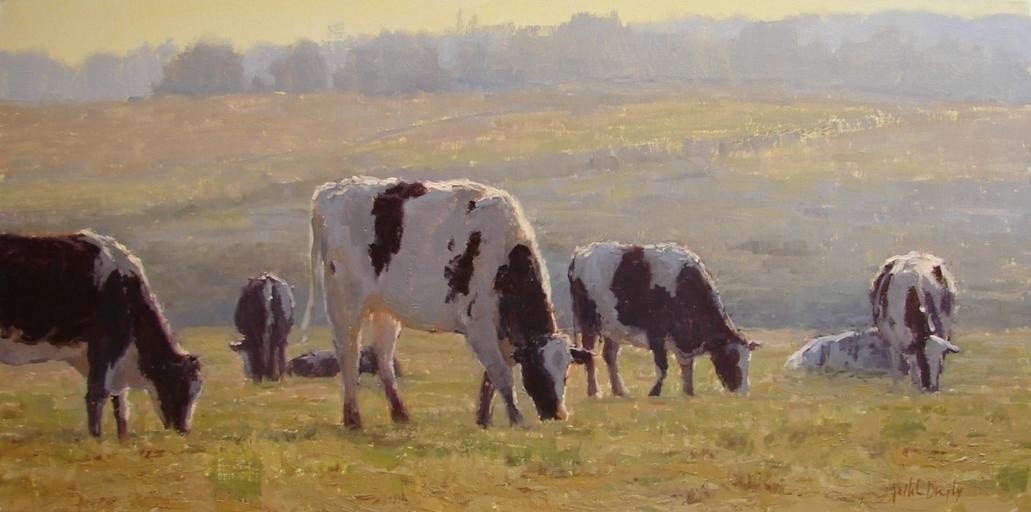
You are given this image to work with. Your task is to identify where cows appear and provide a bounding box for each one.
[868,249,961,394]
[298,175,598,433]
[228,271,296,385]
[0,227,205,448]
[287,345,402,379]
[780,326,912,378]
[567,240,765,398]
